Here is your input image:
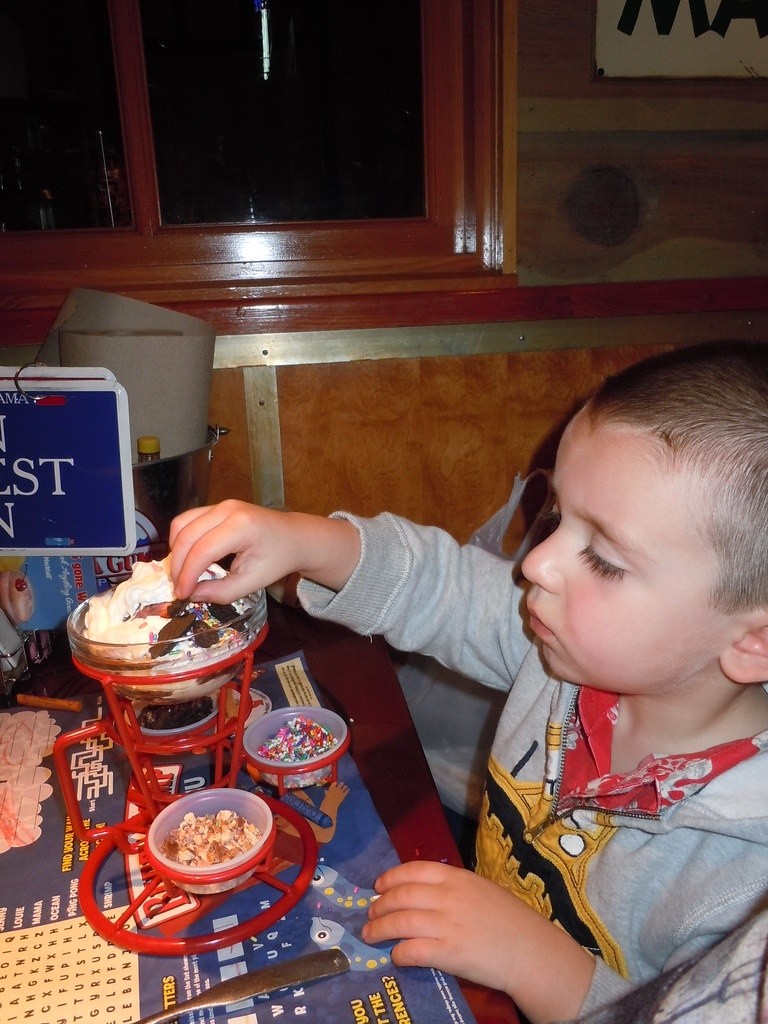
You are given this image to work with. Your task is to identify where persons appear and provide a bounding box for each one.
[170,341,768,1024]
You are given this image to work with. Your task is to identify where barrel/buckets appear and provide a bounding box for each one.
[93,424,232,594]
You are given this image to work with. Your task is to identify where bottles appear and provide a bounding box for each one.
[137,435,162,464]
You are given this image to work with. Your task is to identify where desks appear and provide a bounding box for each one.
[0,602,524,1024]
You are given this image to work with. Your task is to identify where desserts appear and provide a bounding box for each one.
[84,552,255,700]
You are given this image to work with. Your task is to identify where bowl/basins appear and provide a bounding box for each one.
[147,788,273,895]
[128,702,218,738]
[66,577,268,704]
[243,705,347,789]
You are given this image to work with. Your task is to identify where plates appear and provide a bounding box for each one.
[229,688,272,739]
